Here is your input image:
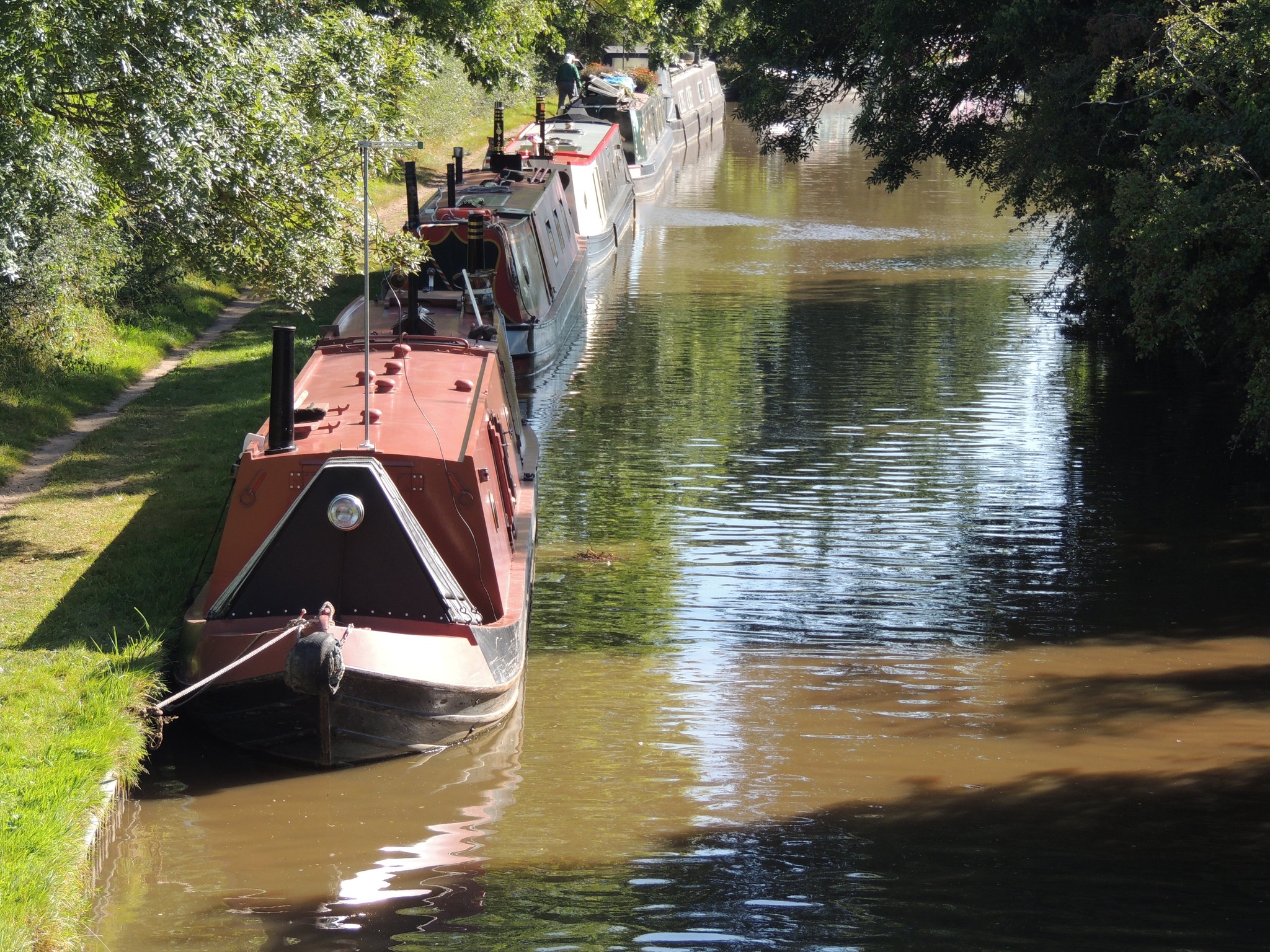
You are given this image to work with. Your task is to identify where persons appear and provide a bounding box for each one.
[556,53,582,115]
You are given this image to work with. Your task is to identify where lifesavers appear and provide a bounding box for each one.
[436,207,491,221]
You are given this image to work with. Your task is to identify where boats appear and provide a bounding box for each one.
[179,273,537,756]
[568,43,730,203]
[392,162,589,379]
[500,100,636,241]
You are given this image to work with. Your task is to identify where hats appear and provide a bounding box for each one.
[564,54,572,64]
